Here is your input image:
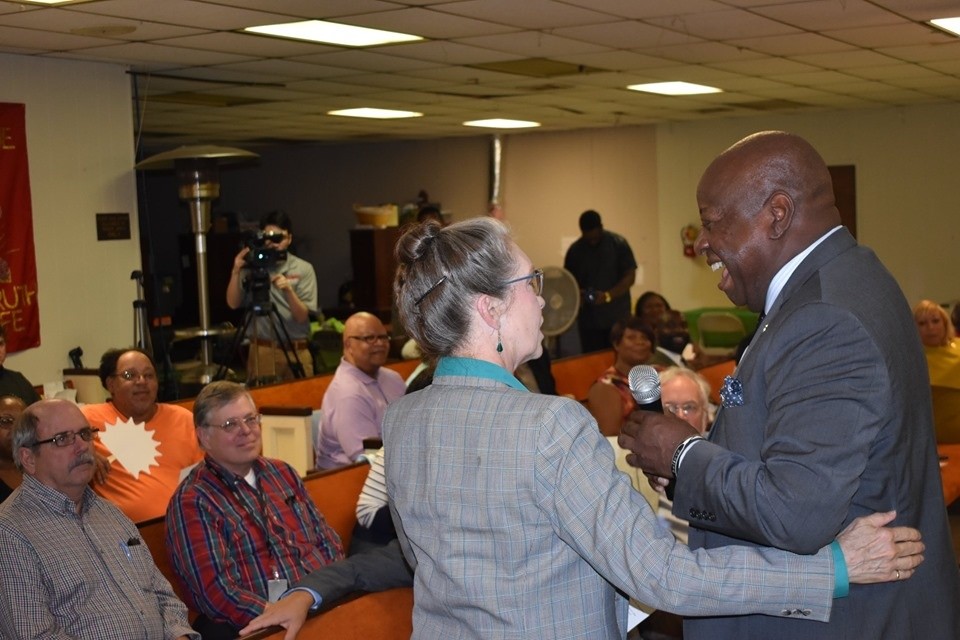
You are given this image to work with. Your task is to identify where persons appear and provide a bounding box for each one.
[77,346,206,609]
[389,206,447,358]
[513,344,559,395]
[0,393,30,505]
[163,379,348,640]
[0,325,43,406]
[355,366,436,542]
[613,362,711,548]
[239,541,415,640]
[314,311,405,473]
[380,215,926,640]
[585,313,712,436]
[563,208,639,355]
[0,399,202,640]
[913,301,960,445]
[616,128,960,640]
[226,208,317,387]
[635,291,737,370]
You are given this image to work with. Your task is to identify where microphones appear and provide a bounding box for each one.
[631,366,678,501]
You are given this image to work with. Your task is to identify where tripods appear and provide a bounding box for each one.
[130,271,154,362]
[210,272,305,385]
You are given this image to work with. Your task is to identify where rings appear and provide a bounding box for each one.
[896,570,899,581]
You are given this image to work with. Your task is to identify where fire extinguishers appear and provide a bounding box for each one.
[681,224,696,257]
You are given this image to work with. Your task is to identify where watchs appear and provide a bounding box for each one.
[604,290,611,302]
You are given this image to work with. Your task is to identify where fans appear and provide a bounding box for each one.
[540,265,580,348]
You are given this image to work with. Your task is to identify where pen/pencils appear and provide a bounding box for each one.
[120,541,133,560]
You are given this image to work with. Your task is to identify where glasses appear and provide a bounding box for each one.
[665,401,699,413]
[35,426,101,447]
[206,413,264,432]
[348,333,391,343]
[499,269,543,296]
[112,367,155,380]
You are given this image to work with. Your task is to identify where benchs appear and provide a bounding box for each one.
[137,352,960,640]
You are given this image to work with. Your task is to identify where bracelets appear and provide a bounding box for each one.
[670,435,707,479]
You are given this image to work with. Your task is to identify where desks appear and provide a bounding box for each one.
[683,307,761,358]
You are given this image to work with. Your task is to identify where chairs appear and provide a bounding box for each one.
[695,312,746,355]
